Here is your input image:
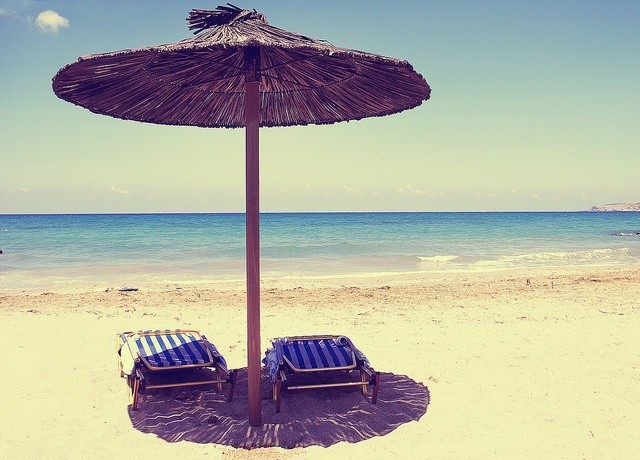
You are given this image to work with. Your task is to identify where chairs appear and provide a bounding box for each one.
[114,329,237,412]
[265,333,379,412]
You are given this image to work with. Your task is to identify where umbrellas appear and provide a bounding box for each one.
[51,1,432,426]
